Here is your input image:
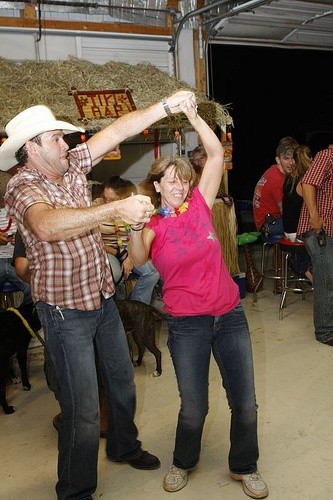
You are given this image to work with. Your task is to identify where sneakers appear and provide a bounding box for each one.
[164,462,199,492]
[230,467,269,499]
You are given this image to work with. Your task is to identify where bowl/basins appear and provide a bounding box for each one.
[288,233,296,242]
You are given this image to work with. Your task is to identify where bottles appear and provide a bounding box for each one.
[198,142,201,149]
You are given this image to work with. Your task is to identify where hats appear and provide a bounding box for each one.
[0,104,86,171]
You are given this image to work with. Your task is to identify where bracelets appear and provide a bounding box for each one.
[130,223,146,231]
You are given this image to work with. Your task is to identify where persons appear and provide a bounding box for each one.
[0,92,197,500]
[255,138,333,344]
[130,98,267,497]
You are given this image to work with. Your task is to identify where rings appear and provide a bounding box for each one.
[161,97,172,116]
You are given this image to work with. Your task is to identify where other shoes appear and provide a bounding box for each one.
[325,339,333,347]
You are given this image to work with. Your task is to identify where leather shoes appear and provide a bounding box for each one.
[114,448,161,470]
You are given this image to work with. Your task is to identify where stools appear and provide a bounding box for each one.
[0,282,22,311]
[253,234,314,320]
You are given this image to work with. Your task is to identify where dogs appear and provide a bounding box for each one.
[0,292,168,414]
